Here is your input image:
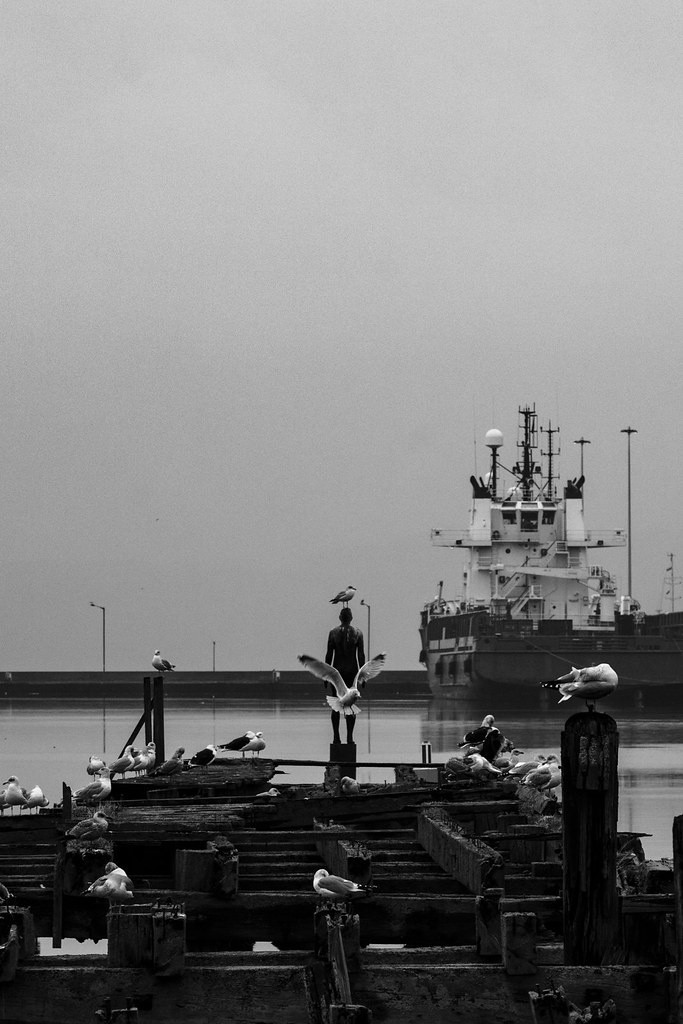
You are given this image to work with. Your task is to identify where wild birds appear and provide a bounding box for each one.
[340,776,359,793]
[80,862,134,899]
[329,585,357,608]
[27,785,49,815]
[65,811,112,840]
[297,653,387,715]
[87,731,266,782]
[457,715,561,794]
[3,776,27,816]
[312,869,378,896]
[540,663,619,712]
[256,788,281,797]
[152,649,175,673]
[73,767,112,809]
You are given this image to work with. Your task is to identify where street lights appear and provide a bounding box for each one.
[90,602,106,672]
[360,602,371,663]
[212,641,216,672]
[573,436,592,512]
[619,425,638,598]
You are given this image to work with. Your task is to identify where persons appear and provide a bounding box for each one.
[324,608,366,745]
[446,607,450,615]
[456,607,460,615]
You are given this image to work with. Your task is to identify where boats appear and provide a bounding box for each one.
[418,397,681,703]
